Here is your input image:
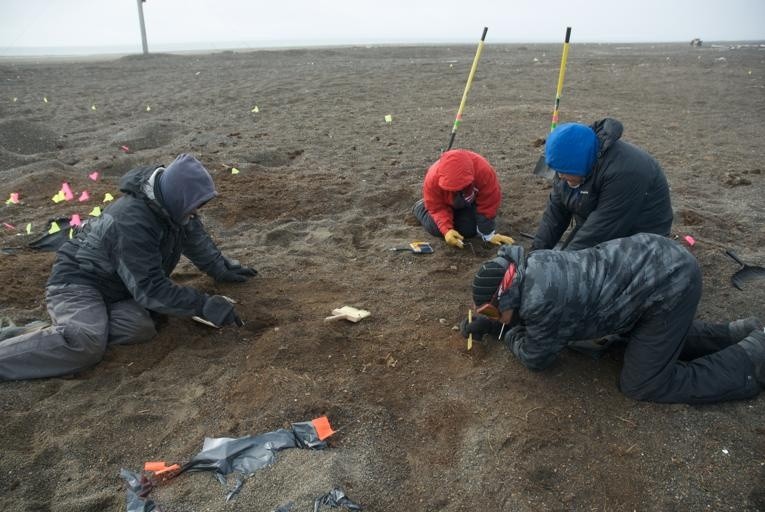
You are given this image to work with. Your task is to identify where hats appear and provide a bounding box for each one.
[473,257,507,307]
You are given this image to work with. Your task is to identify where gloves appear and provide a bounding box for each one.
[489,234,513,244]
[201,295,242,328]
[459,313,508,340]
[219,256,257,282]
[443,230,465,249]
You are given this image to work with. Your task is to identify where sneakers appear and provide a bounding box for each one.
[0,321,50,341]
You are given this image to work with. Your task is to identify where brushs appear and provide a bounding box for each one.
[325,305,371,323]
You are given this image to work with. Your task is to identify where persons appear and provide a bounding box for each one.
[410,148,515,248]
[524,118,675,268]
[459,232,765,402]
[1,152,259,381]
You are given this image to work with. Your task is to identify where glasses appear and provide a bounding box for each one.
[476,263,514,319]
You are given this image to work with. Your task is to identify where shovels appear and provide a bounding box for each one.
[533,27,571,179]
[457,238,476,257]
